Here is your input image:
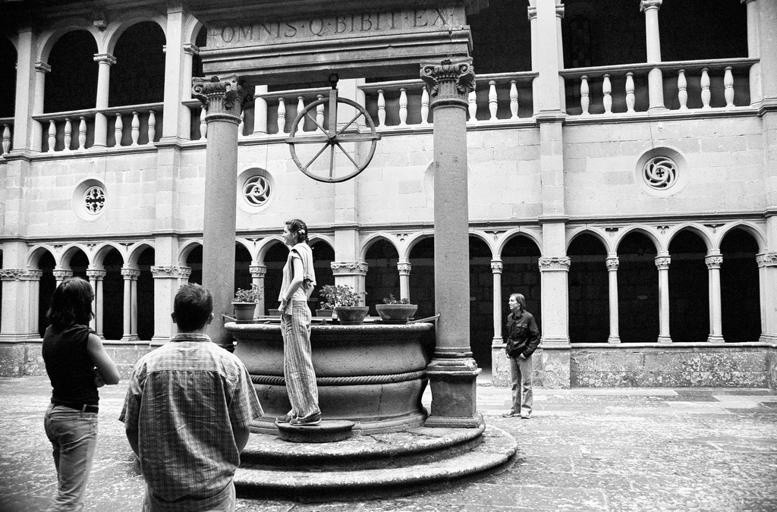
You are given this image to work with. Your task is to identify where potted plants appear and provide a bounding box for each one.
[375,294,418,320]
[231,283,261,321]
[316,285,336,316]
[336,285,369,324]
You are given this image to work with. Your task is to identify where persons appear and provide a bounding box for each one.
[43,277,120,512]
[276,219,322,425]
[502,293,540,419]
[119,283,265,512]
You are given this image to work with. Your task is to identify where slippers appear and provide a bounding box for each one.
[276,411,321,425]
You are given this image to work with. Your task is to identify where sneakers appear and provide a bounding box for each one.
[502,407,531,419]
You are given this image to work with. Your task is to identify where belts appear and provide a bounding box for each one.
[50,398,98,412]
